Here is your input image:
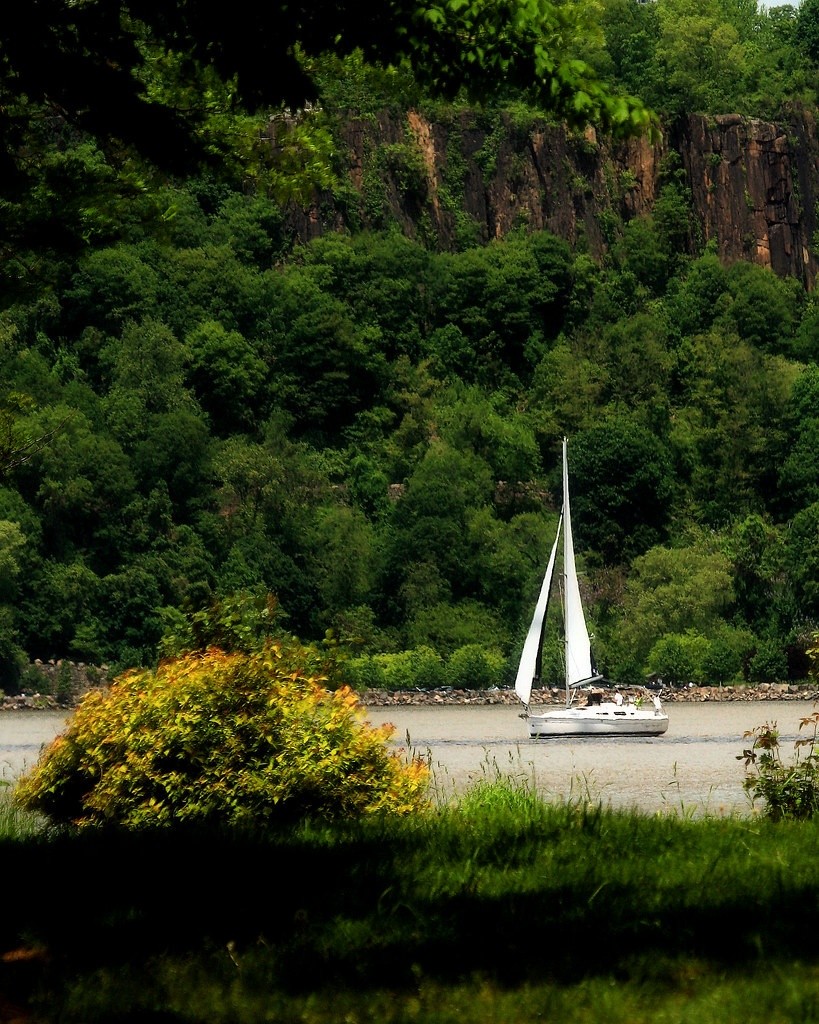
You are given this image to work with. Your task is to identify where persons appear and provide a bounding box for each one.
[634,693,643,710]
[614,690,623,706]
[654,694,661,716]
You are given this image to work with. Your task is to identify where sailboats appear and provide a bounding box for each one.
[515,436,669,739]
